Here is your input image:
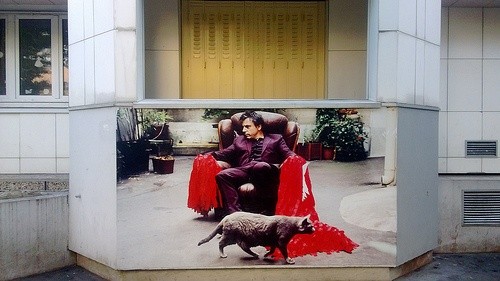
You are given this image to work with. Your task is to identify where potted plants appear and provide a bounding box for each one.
[142,110,173,140]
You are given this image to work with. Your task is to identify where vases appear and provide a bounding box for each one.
[152,156,175,174]
[323,145,332,160]
[298,142,322,161]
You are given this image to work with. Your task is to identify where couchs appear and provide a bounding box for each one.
[201,111,300,219]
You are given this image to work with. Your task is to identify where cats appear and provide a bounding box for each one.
[197,211,315,265]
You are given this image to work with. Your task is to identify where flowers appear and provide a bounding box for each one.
[303,108,369,161]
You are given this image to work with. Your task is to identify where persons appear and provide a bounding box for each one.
[197,111,304,218]
